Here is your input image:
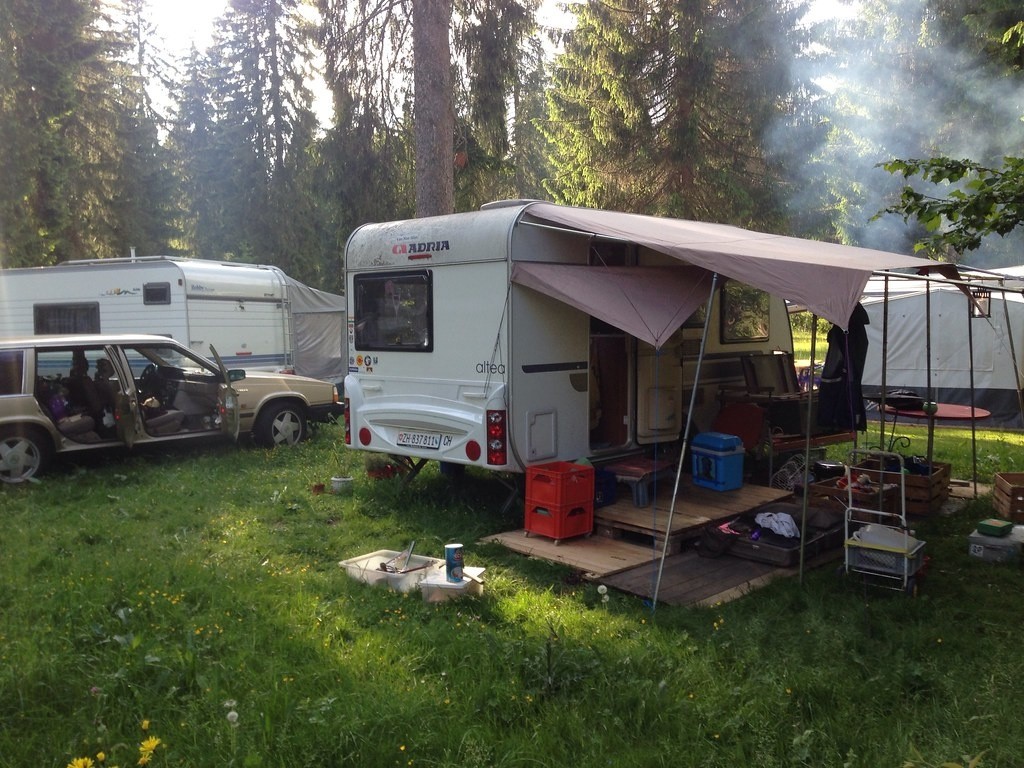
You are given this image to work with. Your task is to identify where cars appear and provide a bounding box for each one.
[0,334,345,487]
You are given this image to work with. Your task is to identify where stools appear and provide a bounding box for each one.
[610,456,677,510]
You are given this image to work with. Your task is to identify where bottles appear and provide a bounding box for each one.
[923,402,937,414]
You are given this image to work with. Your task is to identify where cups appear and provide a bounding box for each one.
[445,543,464,583]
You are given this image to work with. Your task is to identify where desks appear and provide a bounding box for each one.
[877,401,991,498]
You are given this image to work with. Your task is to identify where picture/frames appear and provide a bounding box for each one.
[720,277,773,344]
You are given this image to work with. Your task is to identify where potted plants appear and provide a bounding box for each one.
[330,444,356,498]
[301,470,325,493]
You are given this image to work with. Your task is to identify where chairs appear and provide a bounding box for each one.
[94,357,185,435]
[71,354,114,435]
[797,363,826,390]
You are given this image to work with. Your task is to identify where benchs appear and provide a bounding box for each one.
[753,426,857,490]
[33,395,103,443]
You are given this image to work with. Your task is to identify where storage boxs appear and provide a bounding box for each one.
[797,457,952,577]
[977,519,1014,537]
[338,549,446,593]
[419,565,486,603]
[744,353,830,436]
[991,473,1024,526]
[966,526,1024,564]
[525,460,595,545]
[691,431,748,494]
[595,470,616,508]
